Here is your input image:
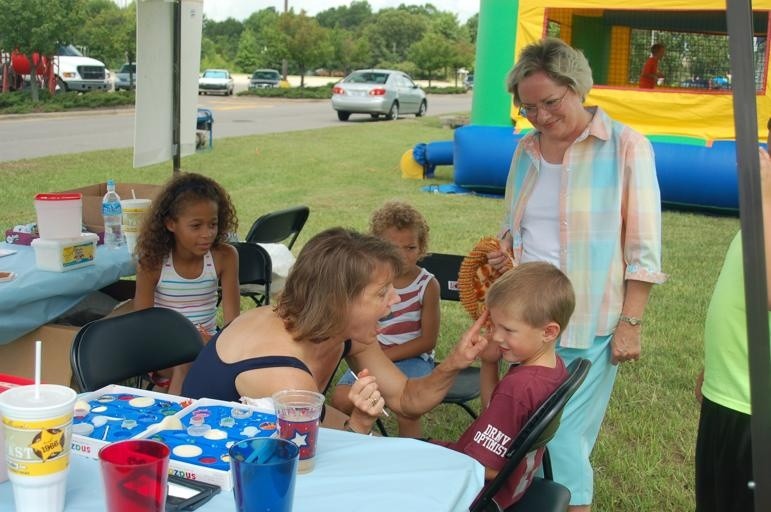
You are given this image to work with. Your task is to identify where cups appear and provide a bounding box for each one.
[98,440,171,512]
[118,199,152,256]
[272,390,325,474]
[0,384,75,512]
[230,437,300,512]
[102,180,122,250]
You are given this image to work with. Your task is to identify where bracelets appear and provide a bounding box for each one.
[345,419,373,435]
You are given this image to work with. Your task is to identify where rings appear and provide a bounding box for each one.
[368,395,376,406]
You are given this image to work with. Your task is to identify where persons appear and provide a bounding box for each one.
[181,225,491,436]
[133,174,240,396]
[332,201,440,440]
[413,261,575,512]
[639,43,665,87]
[695,119,770,510]
[497,36,667,511]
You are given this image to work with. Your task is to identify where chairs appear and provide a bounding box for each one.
[373,252,487,436]
[232,205,308,306]
[462,358,592,512]
[218,242,272,320]
[68,305,207,397]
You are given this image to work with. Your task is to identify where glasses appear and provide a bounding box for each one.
[517,85,570,118]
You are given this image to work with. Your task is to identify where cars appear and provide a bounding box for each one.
[330,69,427,121]
[50,41,280,96]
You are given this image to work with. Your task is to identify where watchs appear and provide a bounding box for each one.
[619,316,641,326]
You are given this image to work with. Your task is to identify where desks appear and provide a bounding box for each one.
[0,237,139,347]
[0,427,485,512]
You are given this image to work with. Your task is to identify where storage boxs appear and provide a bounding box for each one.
[0,293,124,389]
[59,183,167,242]
